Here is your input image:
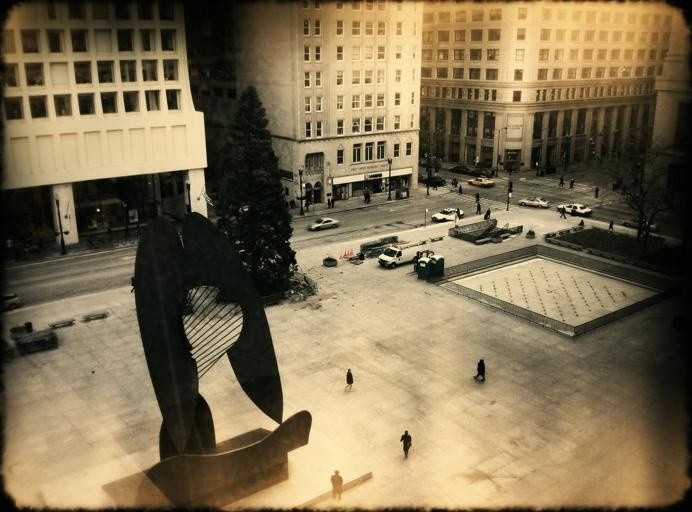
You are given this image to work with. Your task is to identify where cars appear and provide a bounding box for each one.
[2,291,22,311]
[431,207,464,222]
[557,203,593,217]
[419,160,496,189]
[622,216,661,233]
[518,195,551,209]
[307,217,340,231]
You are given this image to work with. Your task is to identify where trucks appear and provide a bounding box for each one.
[377,246,418,269]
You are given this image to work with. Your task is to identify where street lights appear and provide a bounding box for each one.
[54,198,69,256]
[298,167,306,215]
[496,127,508,178]
[387,156,393,201]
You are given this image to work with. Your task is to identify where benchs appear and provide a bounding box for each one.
[48,308,110,329]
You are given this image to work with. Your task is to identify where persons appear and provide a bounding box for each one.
[578,220,585,225]
[558,175,564,187]
[328,198,331,209]
[484,213,489,218]
[473,357,488,381]
[366,191,371,204]
[475,202,481,215]
[475,192,480,204]
[330,470,345,501]
[569,176,576,188]
[345,368,355,391]
[400,428,413,458]
[595,187,599,198]
[485,208,491,218]
[560,205,567,220]
[608,220,614,230]
[330,198,334,207]
[363,193,367,202]
[459,184,463,195]
[456,208,460,223]
